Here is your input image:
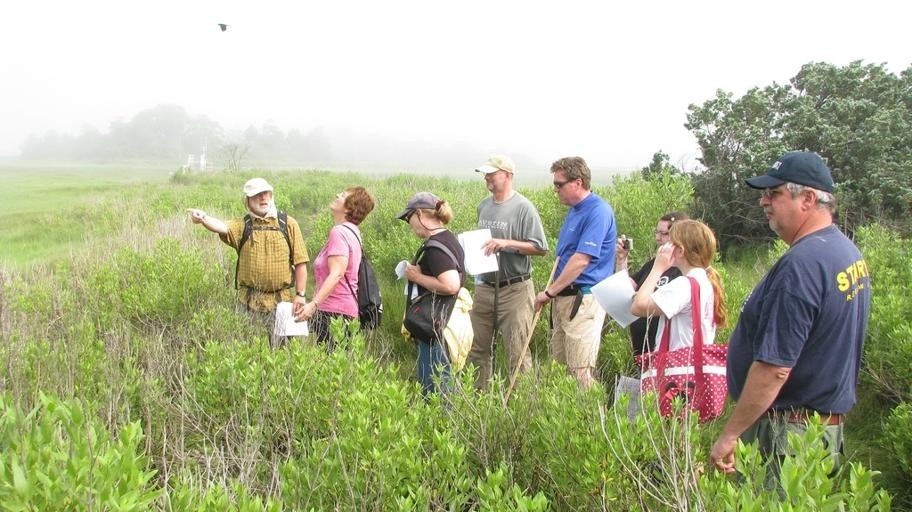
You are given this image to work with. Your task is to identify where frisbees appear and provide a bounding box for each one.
[417,212,420,216]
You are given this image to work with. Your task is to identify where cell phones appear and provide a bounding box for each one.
[623,239,634,251]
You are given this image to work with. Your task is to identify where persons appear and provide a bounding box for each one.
[532,156,618,388]
[632,218,729,484]
[184,176,310,350]
[295,185,376,353]
[709,149,872,502]
[615,212,690,379]
[396,191,465,408]
[462,156,549,389]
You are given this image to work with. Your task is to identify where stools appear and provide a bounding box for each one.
[763,408,845,425]
[558,285,584,321]
[483,273,531,289]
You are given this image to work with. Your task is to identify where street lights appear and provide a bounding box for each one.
[633,342,728,424]
[402,271,463,347]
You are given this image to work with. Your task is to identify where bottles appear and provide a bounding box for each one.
[744,152,836,193]
[475,155,514,175]
[395,192,438,218]
[244,177,272,198]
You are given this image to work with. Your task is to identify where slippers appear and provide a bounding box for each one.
[341,224,383,329]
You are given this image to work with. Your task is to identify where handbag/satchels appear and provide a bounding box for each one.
[544,291,556,300]
[314,300,320,308]
[295,289,306,297]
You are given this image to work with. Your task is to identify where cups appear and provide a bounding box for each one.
[554,179,572,190]
[760,190,789,201]
[403,209,416,224]
